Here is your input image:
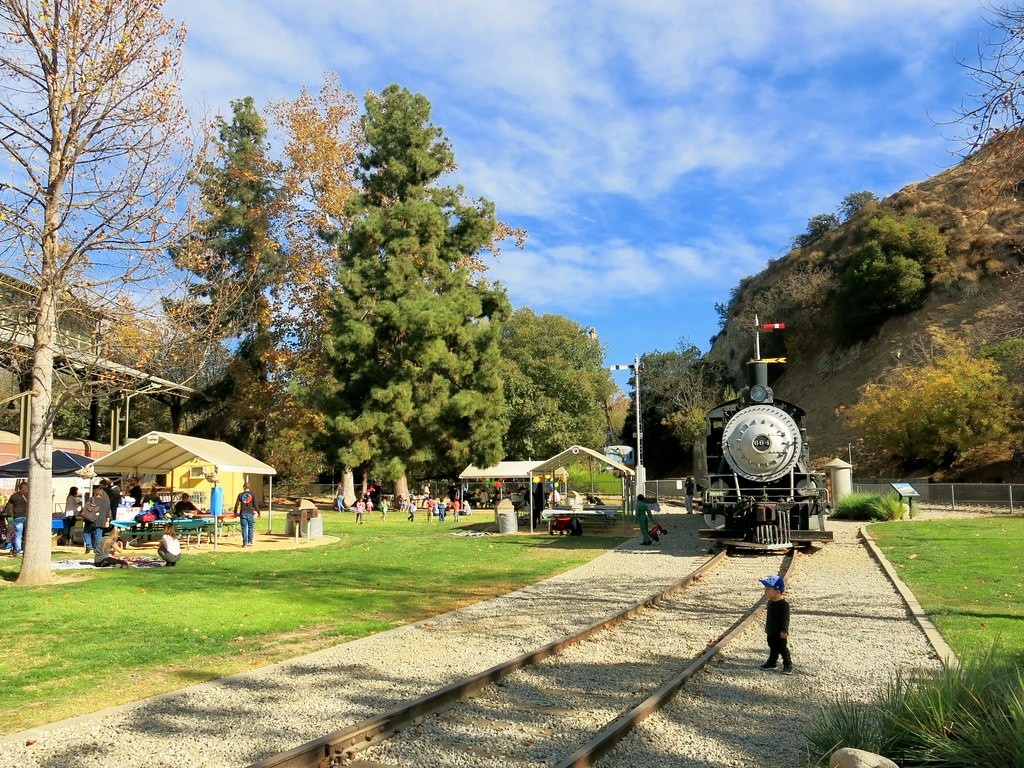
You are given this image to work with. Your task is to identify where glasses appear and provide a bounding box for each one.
[181,497,186,498]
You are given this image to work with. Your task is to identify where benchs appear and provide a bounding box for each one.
[122,522,236,550]
[542,512,623,533]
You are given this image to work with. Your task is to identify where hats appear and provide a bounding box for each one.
[463,501,469,505]
[759,576,784,592]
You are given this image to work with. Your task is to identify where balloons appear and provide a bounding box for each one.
[479,478,515,487]
[461,480,467,488]
[532,474,567,489]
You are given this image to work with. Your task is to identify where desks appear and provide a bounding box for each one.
[110,513,236,542]
[542,506,622,533]
[647,503,661,521]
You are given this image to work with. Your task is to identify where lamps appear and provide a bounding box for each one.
[6,372,15,409]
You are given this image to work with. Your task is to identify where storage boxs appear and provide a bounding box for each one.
[51,535,62,547]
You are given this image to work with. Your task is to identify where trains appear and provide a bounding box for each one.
[693,315,835,554]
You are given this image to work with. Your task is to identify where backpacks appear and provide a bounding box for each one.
[135,510,156,523]
[80,497,99,526]
[569,516,583,536]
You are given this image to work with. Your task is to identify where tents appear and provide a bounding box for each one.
[458,459,568,509]
[529,444,635,533]
[76,430,277,551]
[0,450,98,507]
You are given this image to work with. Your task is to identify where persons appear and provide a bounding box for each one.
[464,487,511,508]
[335,482,345,512]
[94,527,127,568]
[234,483,261,547]
[518,487,539,529]
[355,482,406,524]
[634,494,655,545]
[8,482,29,557]
[684,476,694,514]
[62,477,204,553]
[422,482,461,523]
[536,483,550,523]
[759,576,793,674]
[585,493,606,506]
[408,498,415,522]
[158,524,180,566]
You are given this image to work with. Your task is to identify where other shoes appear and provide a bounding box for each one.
[114,564,127,569]
[166,562,177,567]
[640,539,653,545]
[242,544,247,548]
[85,546,92,555]
[248,541,254,545]
[13,553,20,557]
[526,525,537,529]
[65,541,74,546]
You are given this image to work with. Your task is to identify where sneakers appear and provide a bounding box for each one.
[782,664,793,674]
[760,659,778,670]
